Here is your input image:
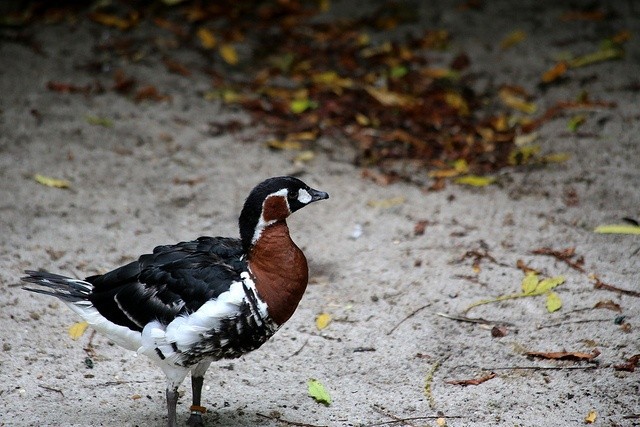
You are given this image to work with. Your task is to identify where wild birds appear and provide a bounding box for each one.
[21,176,329,427]
